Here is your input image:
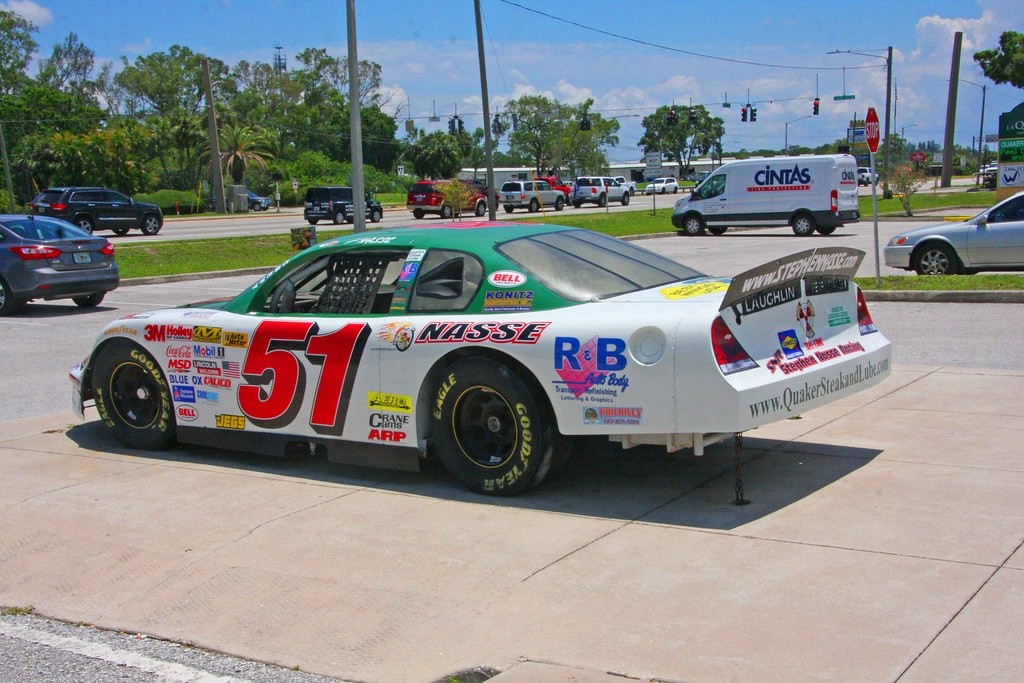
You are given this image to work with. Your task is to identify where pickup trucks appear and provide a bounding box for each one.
[610,175,637,195]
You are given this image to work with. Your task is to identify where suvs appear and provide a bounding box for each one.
[406,180,488,219]
[534,177,572,205]
[211,188,272,211]
[499,180,565,213]
[28,187,166,237]
[569,176,630,208]
[857,167,879,187]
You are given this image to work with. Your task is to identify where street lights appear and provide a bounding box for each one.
[785,115,813,156]
[826,46,893,198]
[900,123,919,165]
[946,79,987,185]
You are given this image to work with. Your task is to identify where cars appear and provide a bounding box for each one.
[645,177,679,196]
[456,179,500,212]
[979,162,997,175]
[688,170,711,186]
[0,214,120,316]
[884,191,1024,277]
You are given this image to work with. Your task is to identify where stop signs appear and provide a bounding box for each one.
[864,107,881,153]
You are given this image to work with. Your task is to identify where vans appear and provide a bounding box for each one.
[303,186,385,226]
[671,153,861,235]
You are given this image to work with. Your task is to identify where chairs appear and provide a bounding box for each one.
[4,226,26,243]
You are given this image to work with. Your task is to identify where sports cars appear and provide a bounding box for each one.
[68,220,895,495]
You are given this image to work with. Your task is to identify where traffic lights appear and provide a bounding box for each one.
[448,119,456,135]
[458,118,465,134]
[813,101,820,115]
[750,107,758,122]
[667,110,679,125]
[689,108,697,121]
[741,108,747,122]
